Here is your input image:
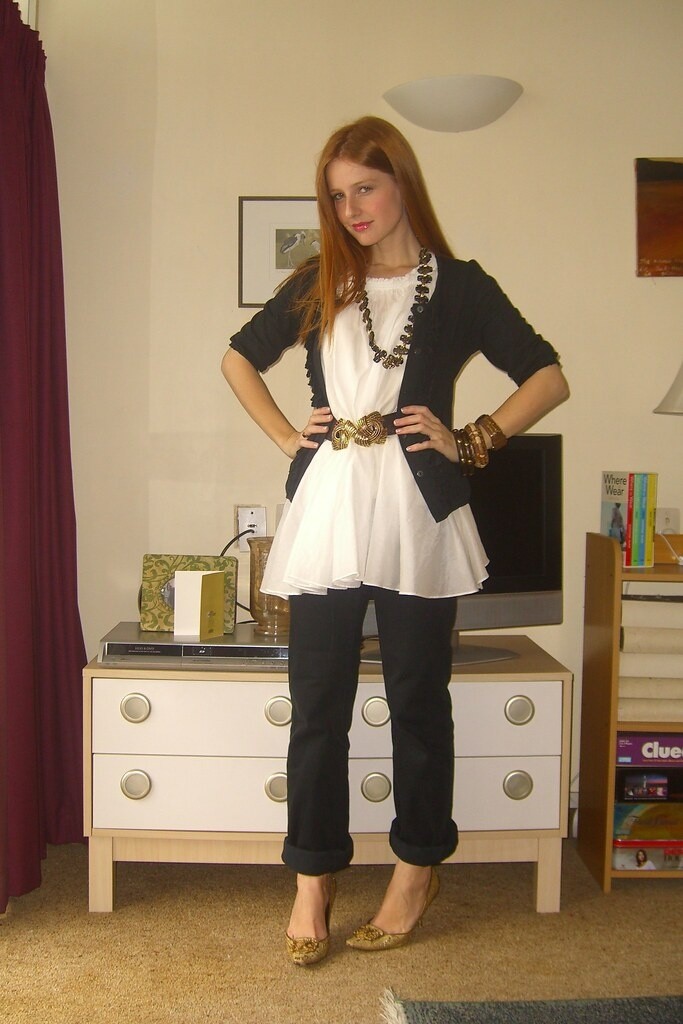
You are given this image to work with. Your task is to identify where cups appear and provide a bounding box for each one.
[248,537,292,636]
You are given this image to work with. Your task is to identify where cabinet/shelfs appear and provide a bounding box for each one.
[78,634,576,913]
[576,532,683,889]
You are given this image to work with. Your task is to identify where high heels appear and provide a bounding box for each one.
[283,878,336,965]
[347,863,440,950]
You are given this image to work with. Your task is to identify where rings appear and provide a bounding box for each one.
[302,430,310,440]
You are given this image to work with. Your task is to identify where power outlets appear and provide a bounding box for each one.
[655,507,681,535]
[238,506,266,551]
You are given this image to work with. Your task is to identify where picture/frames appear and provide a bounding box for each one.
[239,195,318,309]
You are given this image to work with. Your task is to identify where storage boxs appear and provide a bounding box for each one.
[614,802,683,840]
[615,733,683,769]
[621,770,670,801]
[612,840,683,871]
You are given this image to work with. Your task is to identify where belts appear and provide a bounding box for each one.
[326,413,398,452]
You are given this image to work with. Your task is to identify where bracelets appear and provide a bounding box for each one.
[452,417,507,471]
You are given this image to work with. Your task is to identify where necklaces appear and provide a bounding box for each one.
[354,245,432,369]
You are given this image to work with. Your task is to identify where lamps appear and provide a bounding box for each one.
[382,72,524,135]
[653,362,682,414]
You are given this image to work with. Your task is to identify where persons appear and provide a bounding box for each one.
[221,117,570,964]
[634,851,656,871]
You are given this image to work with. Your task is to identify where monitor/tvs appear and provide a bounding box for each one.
[362,433,565,666]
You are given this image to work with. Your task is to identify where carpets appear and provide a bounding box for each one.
[380,986,683,1024]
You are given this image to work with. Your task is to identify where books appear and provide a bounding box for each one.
[600,469,658,568]
